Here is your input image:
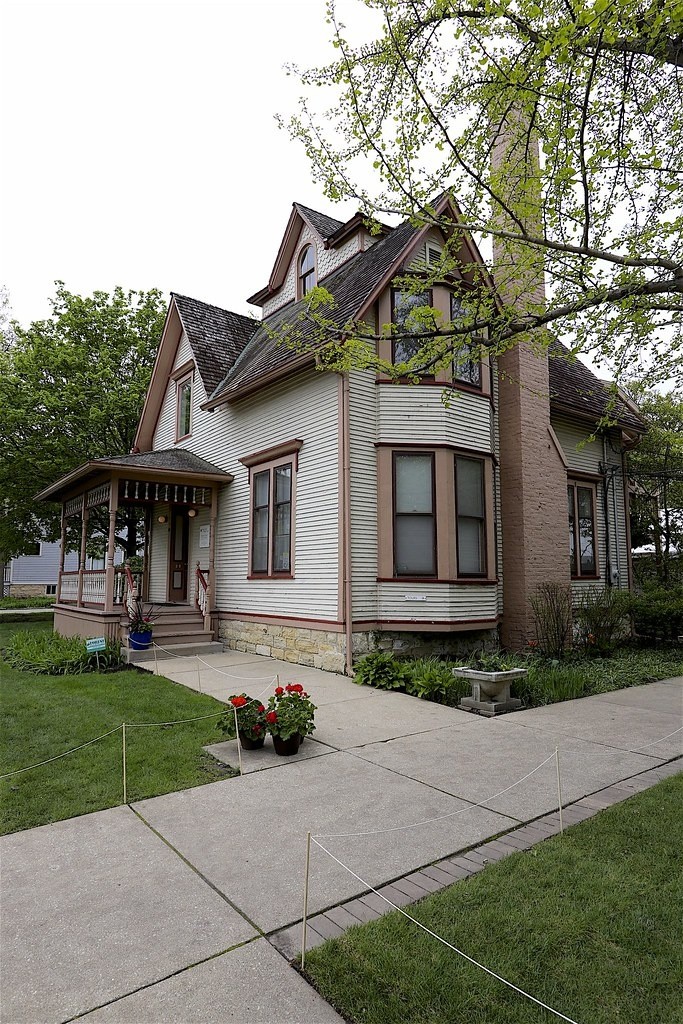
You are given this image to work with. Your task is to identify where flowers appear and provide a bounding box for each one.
[219,684,318,737]
[123,597,164,631]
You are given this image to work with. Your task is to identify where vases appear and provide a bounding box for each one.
[240,726,267,750]
[299,724,306,744]
[271,731,300,756]
[127,629,154,648]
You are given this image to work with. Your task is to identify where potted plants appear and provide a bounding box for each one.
[452,645,527,709]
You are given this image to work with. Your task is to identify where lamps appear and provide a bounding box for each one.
[188,509,198,518]
[156,515,169,523]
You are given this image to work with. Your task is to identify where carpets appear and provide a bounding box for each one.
[145,602,190,607]
